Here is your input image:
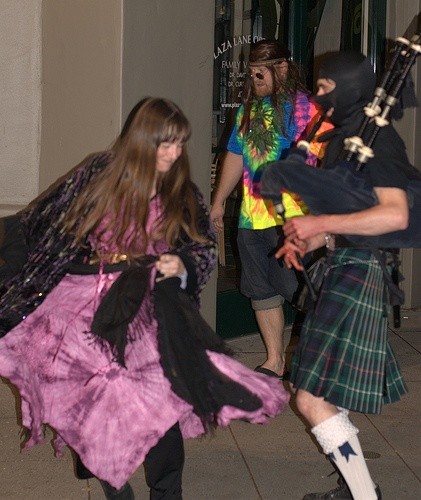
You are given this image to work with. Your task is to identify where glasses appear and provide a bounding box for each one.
[245,68,273,79]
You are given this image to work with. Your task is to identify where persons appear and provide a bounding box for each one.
[0,95,219,500]
[210,39,326,379]
[274,48,412,500]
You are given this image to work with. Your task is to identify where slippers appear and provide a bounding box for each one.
[255,366,291,380]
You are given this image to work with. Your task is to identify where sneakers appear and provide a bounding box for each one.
[302,477,382,500]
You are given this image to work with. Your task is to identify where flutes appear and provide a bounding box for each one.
[293,11,420,174]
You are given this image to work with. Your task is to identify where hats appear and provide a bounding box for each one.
[313,50,377,127]
